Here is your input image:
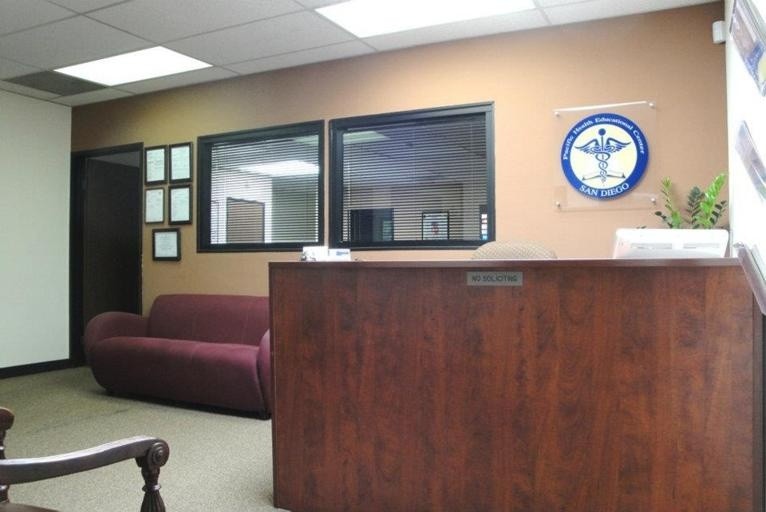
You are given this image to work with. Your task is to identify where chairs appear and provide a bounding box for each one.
[0,401,174,512]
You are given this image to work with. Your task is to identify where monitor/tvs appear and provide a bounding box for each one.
[612,227,729,259]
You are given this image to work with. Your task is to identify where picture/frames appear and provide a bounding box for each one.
[168,184,192,225]
[421,209,449,241]
[152,228,182,261]
[380,216,393,241]
[168,141,193,184]
[144,187,165,223]
[144,144,166,185]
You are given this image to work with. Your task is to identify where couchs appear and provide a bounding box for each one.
[83,293,272,421]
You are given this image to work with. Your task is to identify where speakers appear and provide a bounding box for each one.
[712,21,725,44]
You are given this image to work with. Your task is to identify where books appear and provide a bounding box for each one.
[727,0,766,97]
[737,248,766,316]
[734,121,766,198]
